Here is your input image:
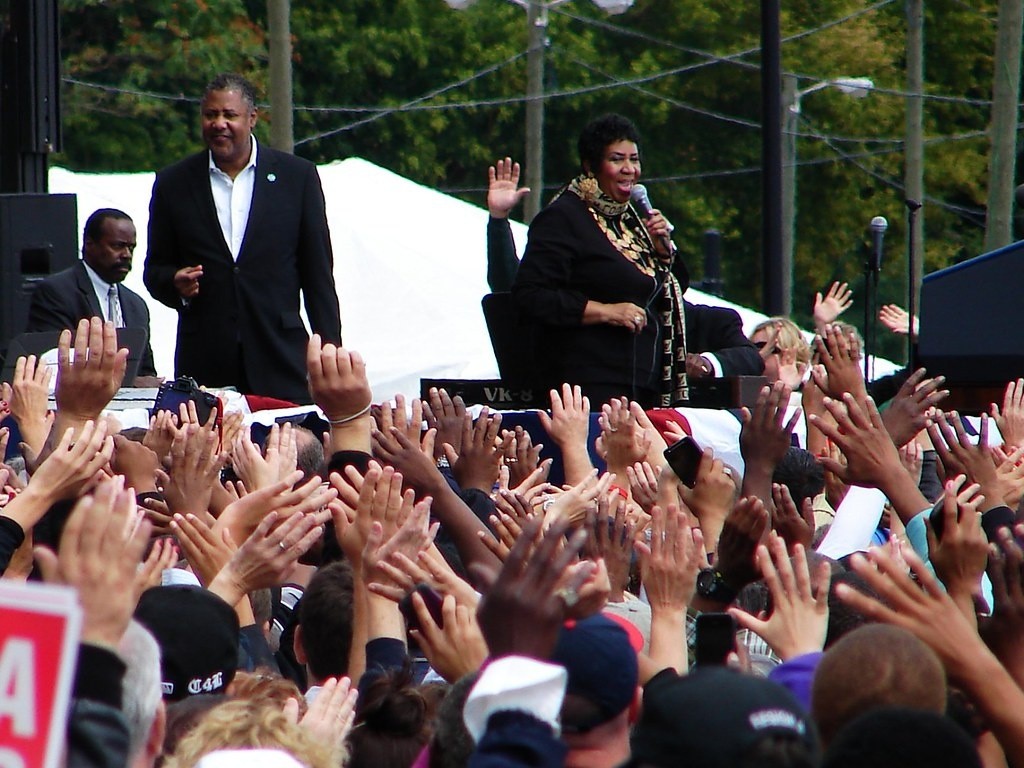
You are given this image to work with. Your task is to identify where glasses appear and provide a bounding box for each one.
[751,342,783,355]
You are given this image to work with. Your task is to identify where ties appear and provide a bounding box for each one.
[108,286,119,328]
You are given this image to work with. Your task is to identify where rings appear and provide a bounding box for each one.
[633,316,641,324]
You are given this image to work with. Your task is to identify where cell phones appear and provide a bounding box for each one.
[662,435,704,488]
[398,581,444,643]
[695,614,737,670]
[930,496,962,544]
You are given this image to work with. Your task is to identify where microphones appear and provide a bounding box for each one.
[871,216,887,286]
[630,184,671,254]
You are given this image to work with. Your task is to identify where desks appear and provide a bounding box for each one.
[459,404,1003,481]
[42,388,333,450]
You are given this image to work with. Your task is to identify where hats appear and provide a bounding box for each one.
[768,649,832,719]
[135,584,241,700]
[631,670,819,768]
[553,609,644,731]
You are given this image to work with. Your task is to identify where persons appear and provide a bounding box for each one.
[23,207,166,388]
[747,279,854,393]
[486,156,531,292]
[810,302,921,409]
[142,74,343,407]
[512,112,678,413]
[669,250,766,380]
[1,323,1024,768]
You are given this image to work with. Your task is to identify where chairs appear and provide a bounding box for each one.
[0,325,148,395]
[483,292,534,406]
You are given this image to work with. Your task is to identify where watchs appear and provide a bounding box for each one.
[655,239,678,259]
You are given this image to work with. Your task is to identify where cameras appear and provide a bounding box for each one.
[152,376,218,431]
